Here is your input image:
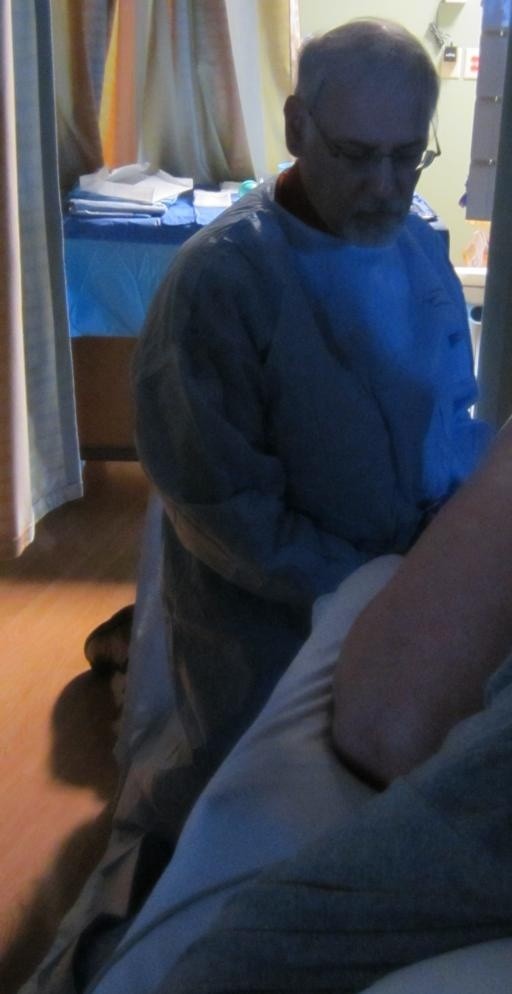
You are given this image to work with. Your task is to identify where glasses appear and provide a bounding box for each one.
[307,116,439,171]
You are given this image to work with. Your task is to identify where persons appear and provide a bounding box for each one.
[155,409,512,992]
[130,16,497,789]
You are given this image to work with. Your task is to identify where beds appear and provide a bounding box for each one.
[77,552,512,993]
